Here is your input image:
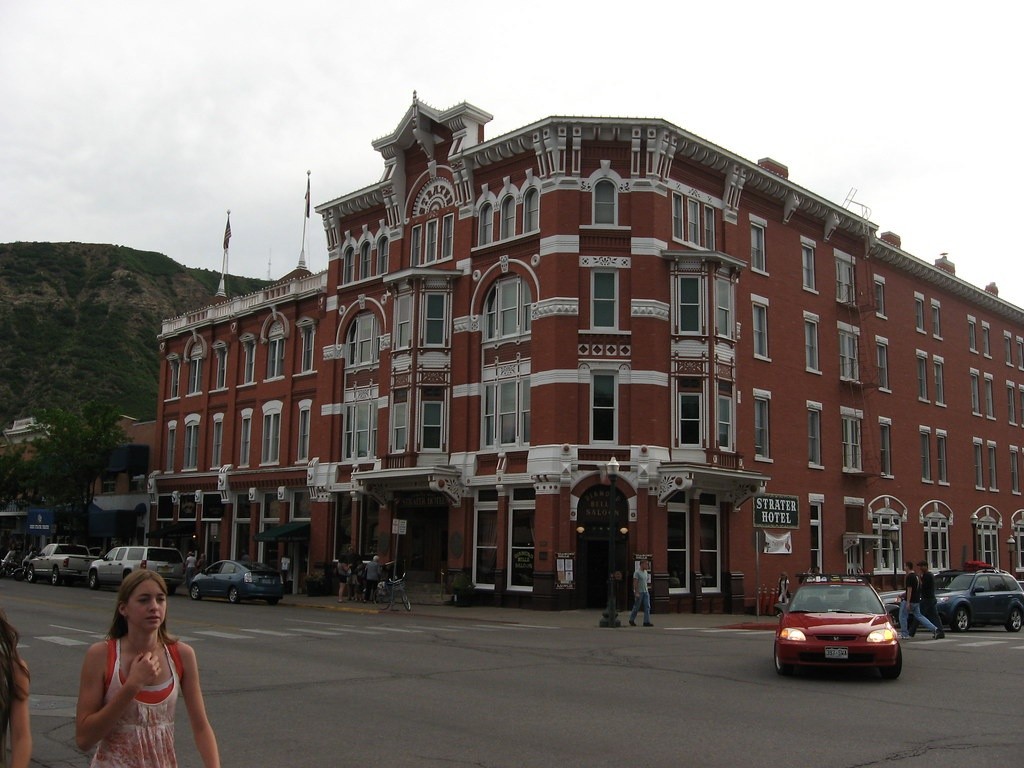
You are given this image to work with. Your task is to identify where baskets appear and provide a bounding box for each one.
[392,581,407,591]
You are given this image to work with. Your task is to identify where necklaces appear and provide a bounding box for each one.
[126,636,157,654]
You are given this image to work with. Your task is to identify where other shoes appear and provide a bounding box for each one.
[933,634,945,638]
[908,633,914,637]
[643,623,653,626]
[935,628,939,639]
[899,633,911,639]
[629,621,636,626]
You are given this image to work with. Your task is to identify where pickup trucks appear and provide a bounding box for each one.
[27,543,100,586]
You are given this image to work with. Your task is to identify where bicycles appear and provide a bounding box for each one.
[373,572,412,611]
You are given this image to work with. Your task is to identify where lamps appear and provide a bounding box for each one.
[576,525,585,534]
[621,526,628,534]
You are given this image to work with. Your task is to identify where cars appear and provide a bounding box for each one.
[773,574,903,681]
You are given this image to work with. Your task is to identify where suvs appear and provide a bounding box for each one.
[190,559,281,604]
[875,569,966,628]
[933,569,1024,633]
[86,546,186,595]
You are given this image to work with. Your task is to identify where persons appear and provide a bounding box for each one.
[628,559,654,626]
[0,611,32,768]
[669,571,680,587]
[776,572,791,617]
[186,551,382,603]
[76,569,220,768]
[898,561,945,640]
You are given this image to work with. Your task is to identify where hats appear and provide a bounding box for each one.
[917,560,928,567]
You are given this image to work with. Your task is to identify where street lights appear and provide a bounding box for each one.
[1006,534,1015,577]
[597,456,621,628]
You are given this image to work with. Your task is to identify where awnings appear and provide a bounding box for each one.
[253,521,311,542]
[103,443,149,473]
[305,179,310,218]
[145,523,196,538]
[223,219,231,249]
[26,505,138,538]
